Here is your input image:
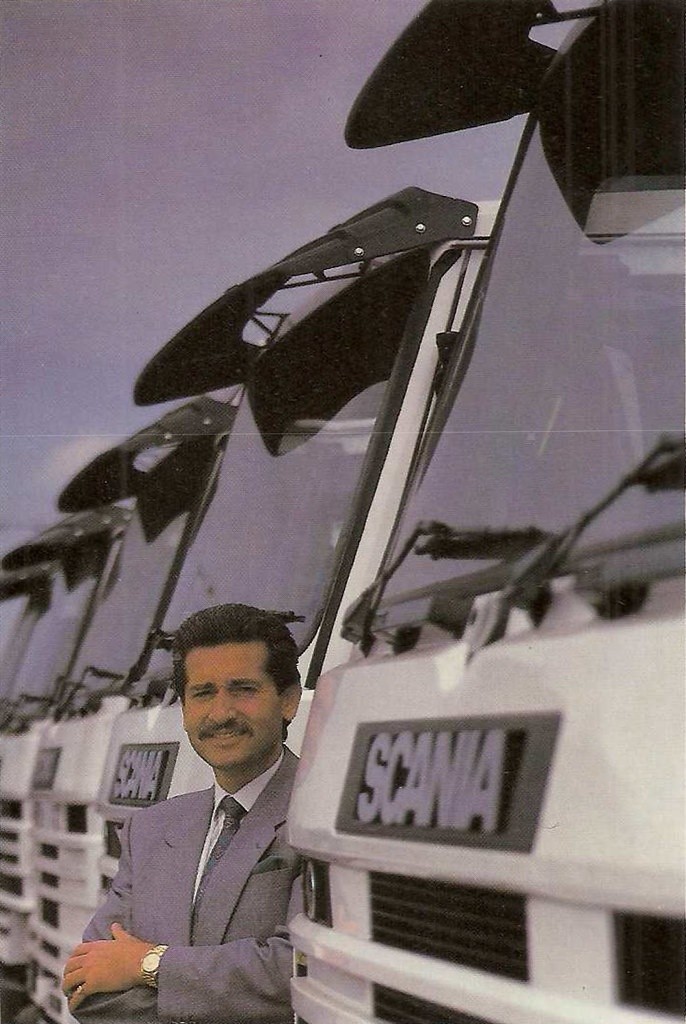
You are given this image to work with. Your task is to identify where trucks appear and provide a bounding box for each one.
[1,185,501,1024]
[286,0,685,1024]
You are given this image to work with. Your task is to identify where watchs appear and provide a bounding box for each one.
[139,944,169,990]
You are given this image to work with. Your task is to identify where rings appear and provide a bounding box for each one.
[75,985,89,999]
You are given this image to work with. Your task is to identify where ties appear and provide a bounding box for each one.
[191,795,248,949]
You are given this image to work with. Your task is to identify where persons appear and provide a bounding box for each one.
[62,604,315,1024]
